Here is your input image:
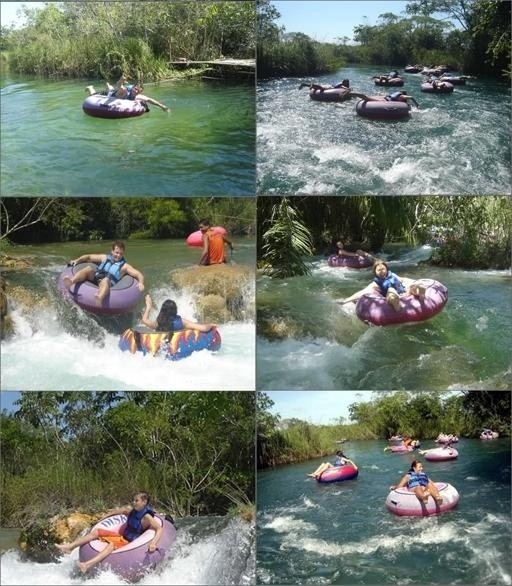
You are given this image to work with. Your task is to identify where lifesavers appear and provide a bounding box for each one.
[83,94,149,117]
[123,327,221,359]
[315,466,357,484]
[188,227,227,246]
[81,513,179,581]
[388,432,498,462]
[326,253,374,270]
[388,482,457,516]
[59,260,143,312]
[307,66,466,119]
[355,278,448,326]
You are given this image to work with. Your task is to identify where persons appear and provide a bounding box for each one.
[369,69,398,83]
[141,293,217,333]
[405,64,476,88]
[62,241,145,305]
[298,79,354,91]
[392,460,443,508]
[85,74,169,112]
[309,451,358,480]
[334,241,380,263]
[198,217,235,265]
[384,430,495,457]
[54,491,163,575]
[344,88,420,109]
[333,259,426,311]
